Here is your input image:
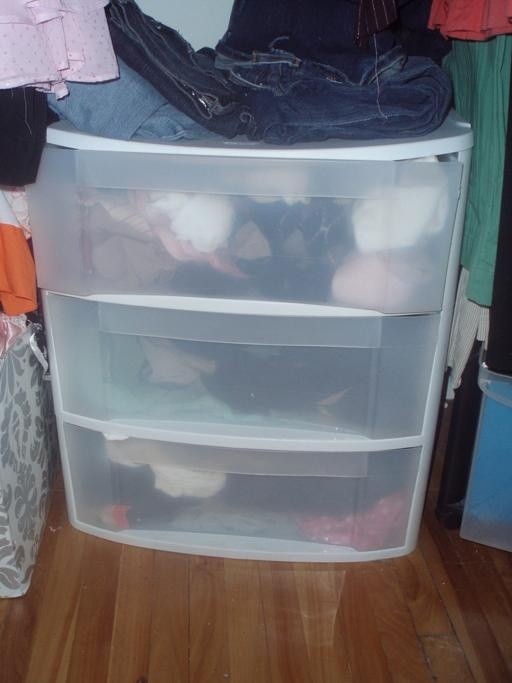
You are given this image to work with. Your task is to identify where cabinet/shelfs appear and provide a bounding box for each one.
[27,115,475,562]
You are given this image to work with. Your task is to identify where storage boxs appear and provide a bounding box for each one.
[0,322,55,598]
[461,343,511,552]
